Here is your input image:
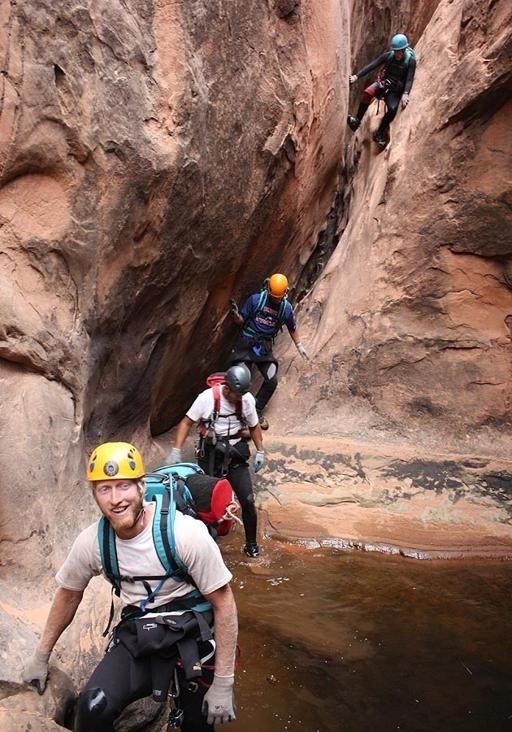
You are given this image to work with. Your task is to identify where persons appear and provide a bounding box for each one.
[224,273,310,431]
[347,33,416,150]
[163,363,266,559]
[23,440,239,732]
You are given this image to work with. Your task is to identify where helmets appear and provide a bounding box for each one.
[268,273,289,297]
[226,366,250,395]
[84,441,146,481]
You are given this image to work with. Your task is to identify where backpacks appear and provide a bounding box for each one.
[251,277,288,332]
[98,460,243,591]
[196,371,247,441]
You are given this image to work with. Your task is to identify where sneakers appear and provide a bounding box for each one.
[244,544,259,558]
[260,417,269,430]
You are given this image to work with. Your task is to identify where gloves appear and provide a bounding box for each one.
[296,342,310,360]
[253,450,265,474]
[201,674,237,725]
[164,447,182,465]
[21,651,51,696]
[230,298,239,317]
